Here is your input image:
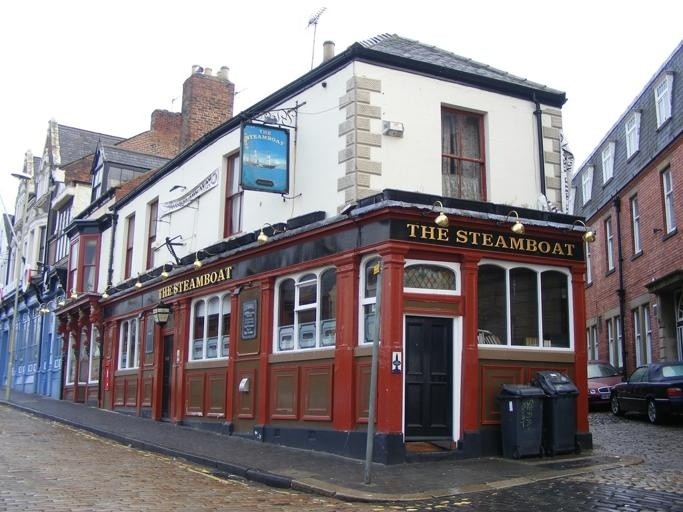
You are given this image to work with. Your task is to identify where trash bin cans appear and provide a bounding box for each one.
[496,370,582,460]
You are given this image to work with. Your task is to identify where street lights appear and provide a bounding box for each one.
[4,171,32,402]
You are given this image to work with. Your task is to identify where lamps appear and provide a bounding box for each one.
[255,221,281,241]
[423,200,596,243]
[151,301,176,330]
[39,248,214,316]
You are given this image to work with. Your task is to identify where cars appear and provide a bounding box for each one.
[608,360,683,425]
[587,359,625,412]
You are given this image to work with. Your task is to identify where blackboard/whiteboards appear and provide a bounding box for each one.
[241,299,257,340]
